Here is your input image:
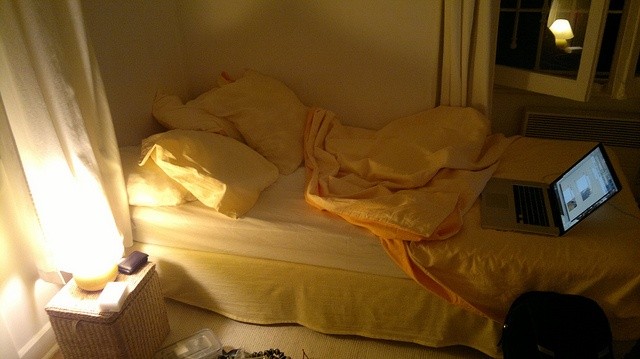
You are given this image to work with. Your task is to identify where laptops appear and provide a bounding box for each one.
[480,143,623,237]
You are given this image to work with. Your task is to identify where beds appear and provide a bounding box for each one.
[129,125,640,359]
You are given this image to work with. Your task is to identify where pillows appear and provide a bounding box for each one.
[118,145,198,206]
[138,129,278,219]
[200,61,309,175]
[152,90,246,143]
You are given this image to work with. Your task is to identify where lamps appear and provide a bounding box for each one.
[56,227,124,293]
[548,1,575,50]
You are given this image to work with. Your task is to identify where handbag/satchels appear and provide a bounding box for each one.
[497,290,614,358]
